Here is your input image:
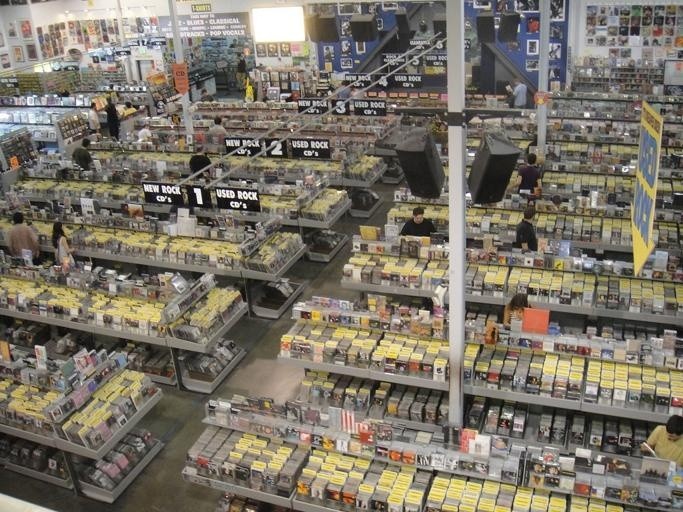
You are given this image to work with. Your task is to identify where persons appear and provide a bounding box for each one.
[139,125,152,143]
[337,80,351,99]
[527,14,538,32]
[507,153,542,195]
[516,205,538,253]
[51,222,76,267]
[504,294,530,330]
[641,414,683,466]
[236,52,247,92]
[124,101,137,116]
[72,139,92,172]
[200,89,214,102]
[210,118,227,134]
[607,37,617,45]
[511,79,528,110]
[57,86,69,97]
[105,96,120,141]
[89,103,101,133]
[190,145,211,179]
[8,212,40,266]
[642,36,649,44]
[400,207,438,237]
[229,39,239,49]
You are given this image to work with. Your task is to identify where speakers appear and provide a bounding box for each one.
[433,11,447,38]
[466,129,522,202]
[497,11,520,44]
[476,11,495,43]
[349,13,377,41]
[394,128,446,199]
[316,13,340,43]
[394,7,411,35]
[304,12,316,42]
